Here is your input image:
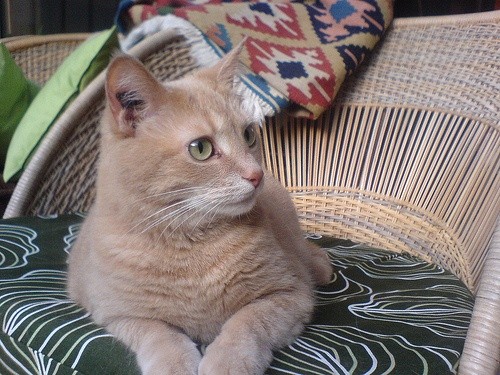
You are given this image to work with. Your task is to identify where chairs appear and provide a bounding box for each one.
[1,9,499,373]
[0,29,114,200]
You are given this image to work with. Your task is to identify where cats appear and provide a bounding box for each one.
[66,35,333,375]
